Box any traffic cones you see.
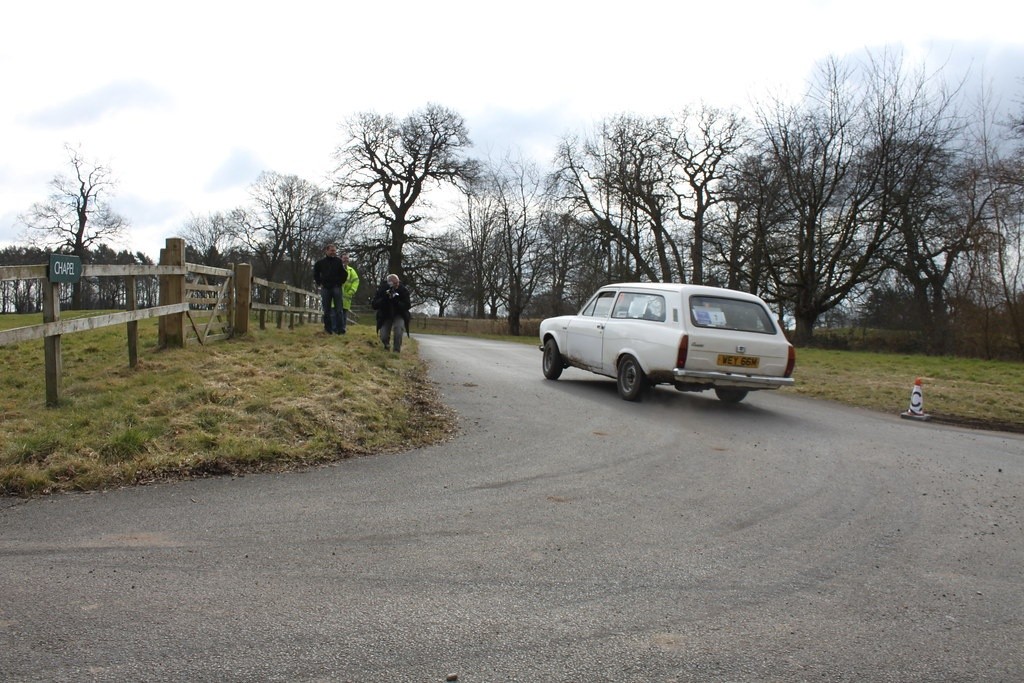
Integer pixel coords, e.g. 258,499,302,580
901,379,932,422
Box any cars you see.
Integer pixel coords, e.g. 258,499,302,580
538,282,799,403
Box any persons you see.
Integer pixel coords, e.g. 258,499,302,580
314,245,348,335
372,273,410,353
330,253,359,330
